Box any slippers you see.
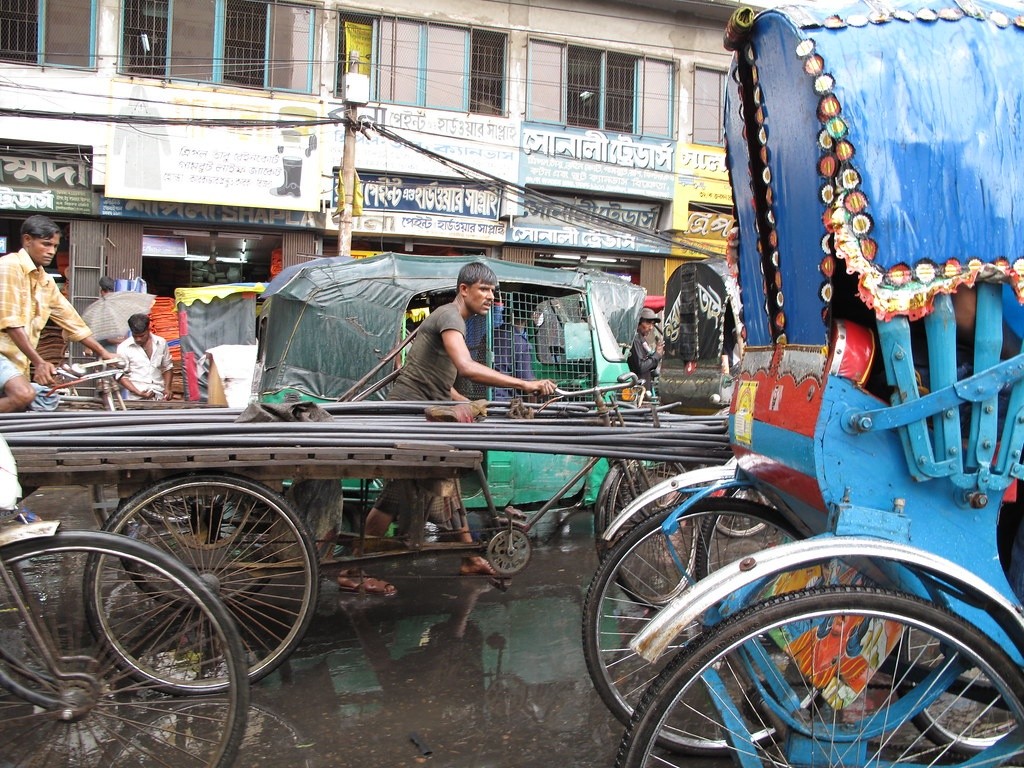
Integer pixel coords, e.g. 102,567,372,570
460,560,497,576
14,507,37,523
339,577,398,595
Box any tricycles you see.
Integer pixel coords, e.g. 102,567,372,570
30,0,1024,767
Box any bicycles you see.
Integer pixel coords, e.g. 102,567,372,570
0,505,252,768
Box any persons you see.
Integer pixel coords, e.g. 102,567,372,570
339,261,557,597
0,215,174,413
626,307,665,392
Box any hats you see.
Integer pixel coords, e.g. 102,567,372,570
642,307,661,324
